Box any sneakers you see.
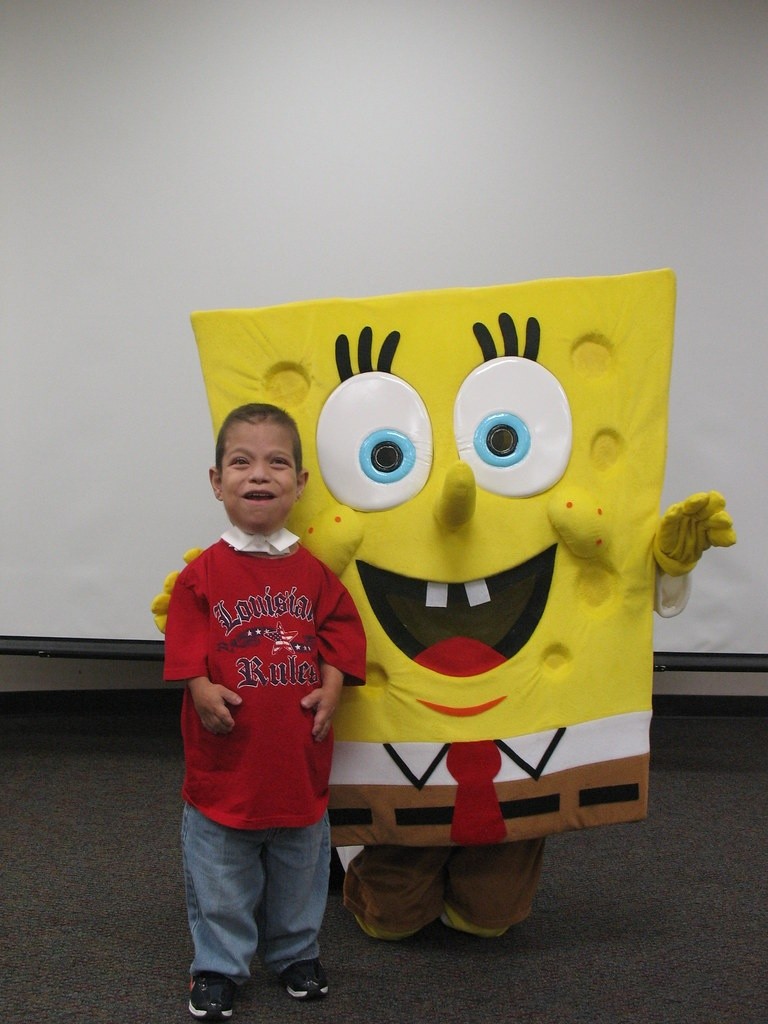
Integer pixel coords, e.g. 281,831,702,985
280,958,329,999
189,972,236,1021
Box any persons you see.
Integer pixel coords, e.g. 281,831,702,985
163,404,367,1018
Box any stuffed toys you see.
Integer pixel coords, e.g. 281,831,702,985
153,268,737,941
